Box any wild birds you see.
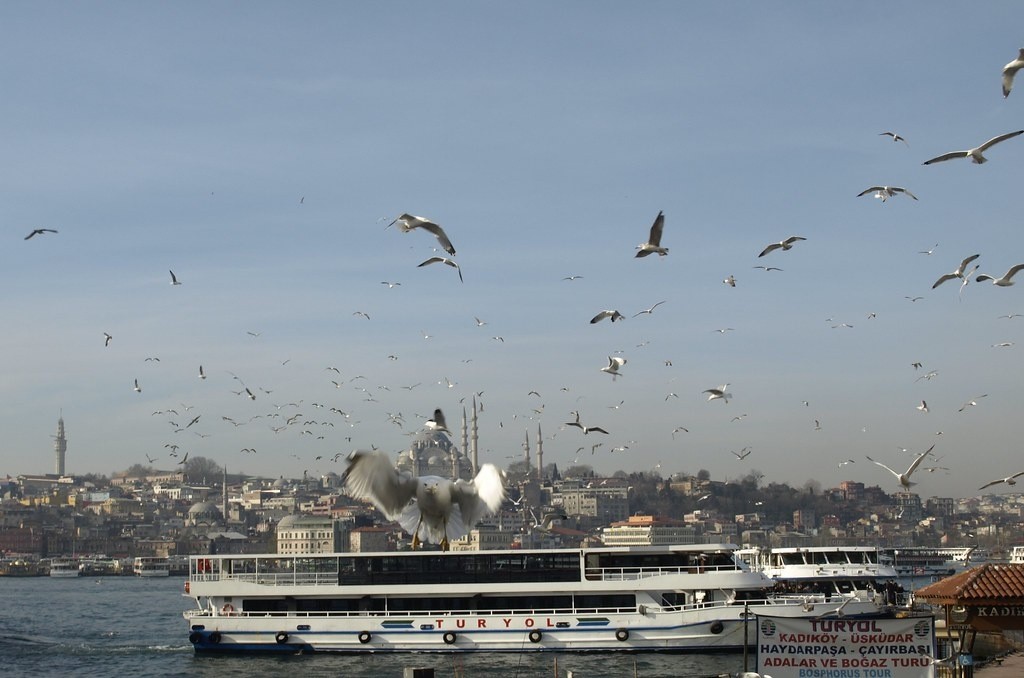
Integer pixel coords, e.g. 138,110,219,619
815,594,857,620
945,545,978,571
104,48,1024,551
23,228,59,240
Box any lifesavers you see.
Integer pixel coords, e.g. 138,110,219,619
616,628,629,642
710,620,723,634
222,604,234,615
357,630,372,644
275,631,287,644
188,631,204,644
198,560,211,572
529,629,542,643
207,632,221,644
443,630,458,645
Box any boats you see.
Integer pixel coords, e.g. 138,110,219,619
181,542,978,659
0,553,248,579
